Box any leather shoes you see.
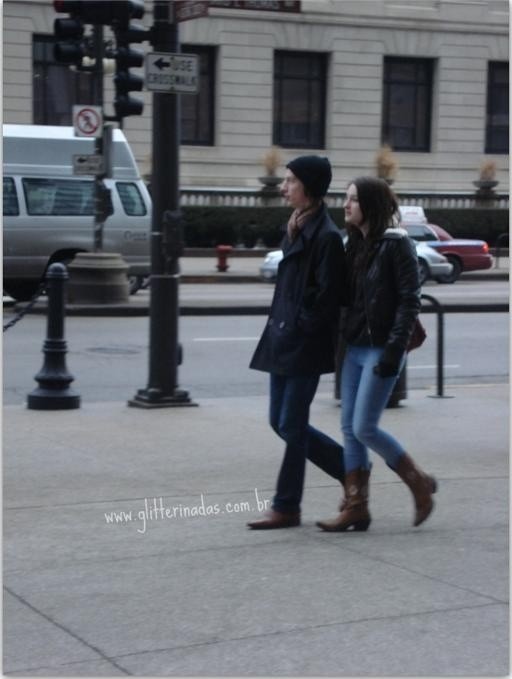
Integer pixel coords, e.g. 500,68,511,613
248,511,300,529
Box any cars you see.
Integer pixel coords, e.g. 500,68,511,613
258,224,454,285
397,205,493,284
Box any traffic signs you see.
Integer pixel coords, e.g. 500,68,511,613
143,52,197,94
72,151,109,178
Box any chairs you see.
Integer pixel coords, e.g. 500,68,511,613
51,189,109,215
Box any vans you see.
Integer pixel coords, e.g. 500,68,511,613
2,120,156,300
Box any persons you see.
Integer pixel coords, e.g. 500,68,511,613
316,177,437,531
244,155,373,529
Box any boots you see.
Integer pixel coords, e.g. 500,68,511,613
317,471,370,530
389,453,435,526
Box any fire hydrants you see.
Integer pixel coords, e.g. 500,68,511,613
215,245,232,272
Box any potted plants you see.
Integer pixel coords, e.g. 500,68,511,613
375,142,397,187
472,157,500,194
261,147,286,191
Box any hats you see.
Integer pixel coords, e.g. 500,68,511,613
287,156,331,197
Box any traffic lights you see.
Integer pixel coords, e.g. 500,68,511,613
114,0,149,117
52,1,84,68
